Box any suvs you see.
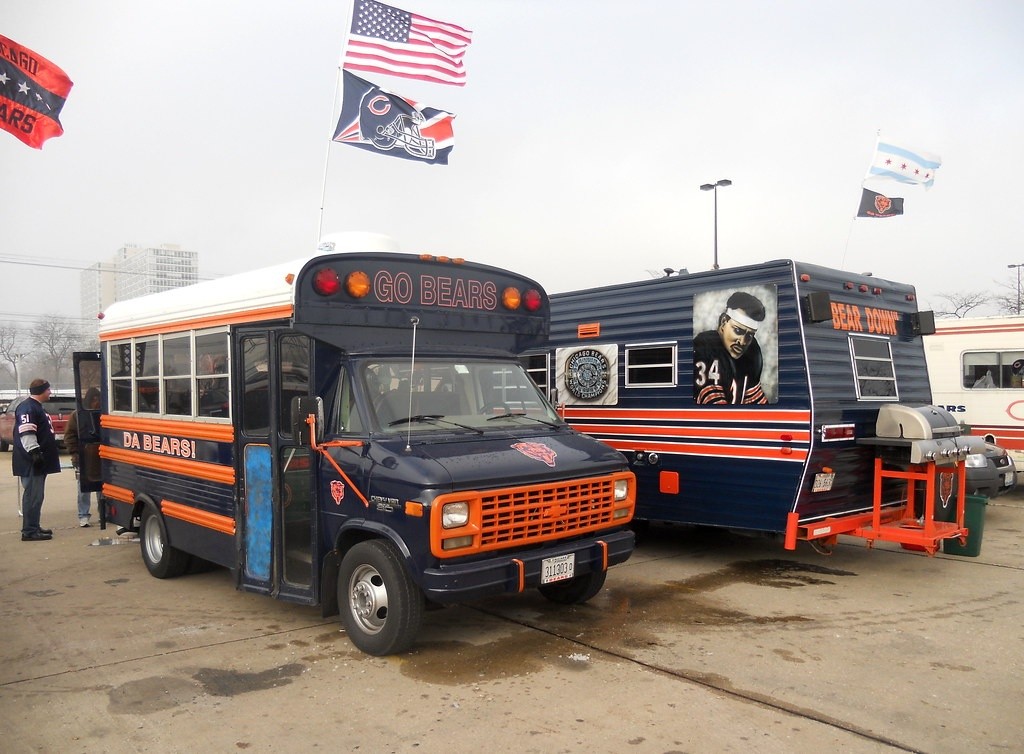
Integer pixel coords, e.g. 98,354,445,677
0,393,77,452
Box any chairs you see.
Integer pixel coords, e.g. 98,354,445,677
372,388,419,433
427,376,464,418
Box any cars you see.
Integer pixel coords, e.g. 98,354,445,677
955,436,1018,501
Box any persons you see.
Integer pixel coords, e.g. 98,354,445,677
64,388,101,527
12,379,61,541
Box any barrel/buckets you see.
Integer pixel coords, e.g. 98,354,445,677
944,495,988,554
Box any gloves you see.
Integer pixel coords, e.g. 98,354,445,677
32,451,45,466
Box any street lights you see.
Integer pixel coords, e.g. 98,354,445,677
700,179,731,270
1008,262,1024,315
15,354,25,398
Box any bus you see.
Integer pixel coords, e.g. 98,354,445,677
69,251,639,656
389,258,984,553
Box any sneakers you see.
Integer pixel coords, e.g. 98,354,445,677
79,517,90,527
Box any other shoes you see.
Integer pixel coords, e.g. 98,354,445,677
22,526,53,541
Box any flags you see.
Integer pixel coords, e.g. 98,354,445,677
0,35,74,151
866,134,943,192
341,0,472,87
330,68,456,165
856,188,905,218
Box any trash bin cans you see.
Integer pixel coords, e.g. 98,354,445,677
943,494,987,558
